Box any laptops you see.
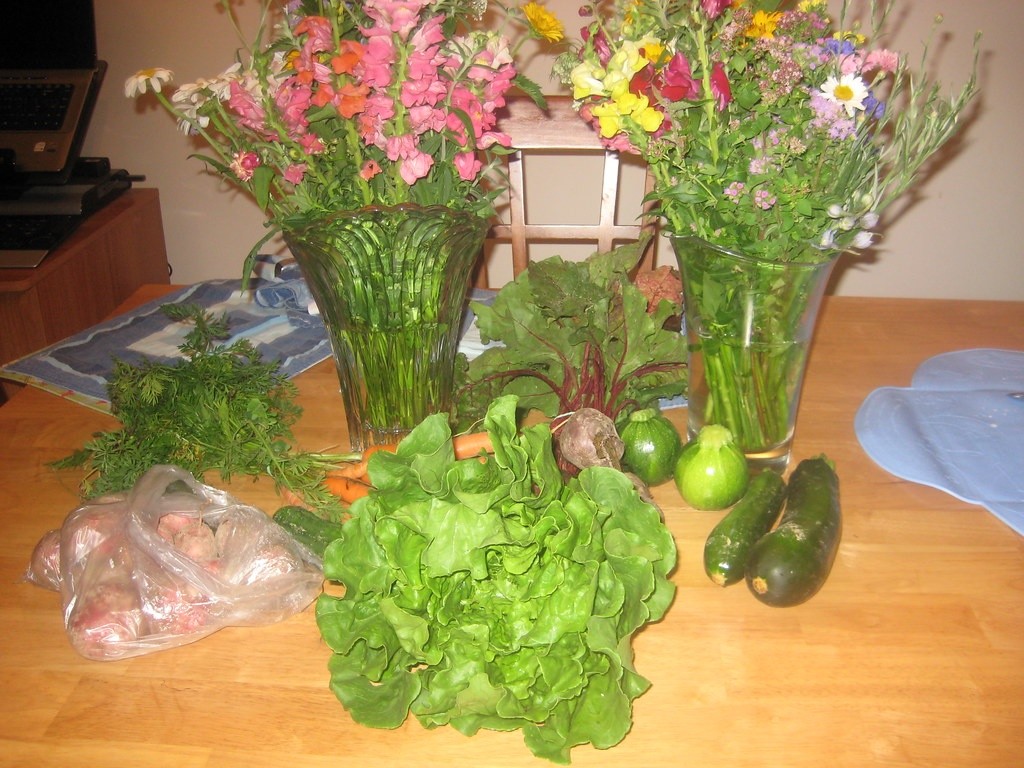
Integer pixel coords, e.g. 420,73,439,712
0,0,99,172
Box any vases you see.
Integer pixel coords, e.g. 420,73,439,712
283,204,491,458
669,235,842,475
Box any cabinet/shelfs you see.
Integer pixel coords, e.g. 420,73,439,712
0,188,171,405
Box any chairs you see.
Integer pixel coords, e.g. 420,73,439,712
460,95,662,289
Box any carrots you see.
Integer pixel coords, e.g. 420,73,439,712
360,430,495,461
323,459,369,479
318,475,372,504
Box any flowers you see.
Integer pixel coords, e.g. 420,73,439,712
122,0,565,435
550,0,995,453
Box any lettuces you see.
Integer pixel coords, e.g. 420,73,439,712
313,391,677,766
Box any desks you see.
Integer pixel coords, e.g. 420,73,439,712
0,281,1024,768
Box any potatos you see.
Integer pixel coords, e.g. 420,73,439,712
26,494,300,661
